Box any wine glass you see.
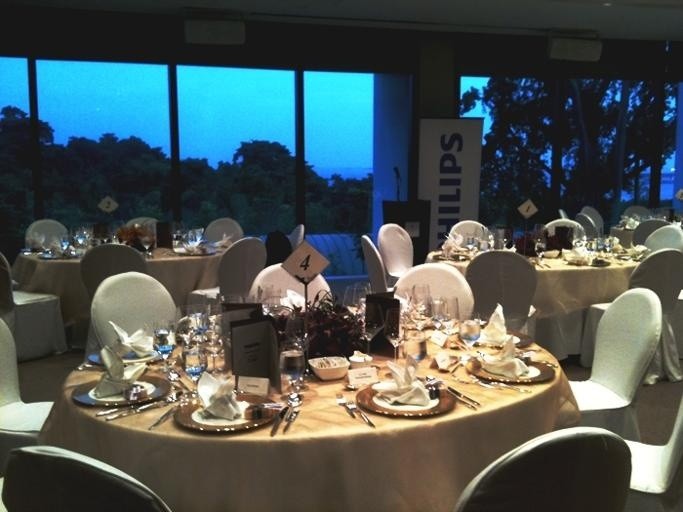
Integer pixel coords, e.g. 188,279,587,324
534,237,545,263
605,234,614,255
151,281,482,407
466,234,475,252
60,224,204,258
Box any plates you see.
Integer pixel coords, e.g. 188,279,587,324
71,330,559,437
